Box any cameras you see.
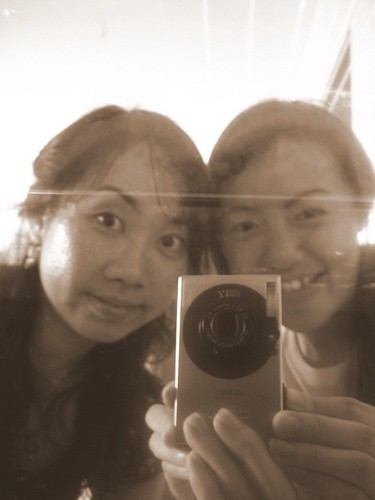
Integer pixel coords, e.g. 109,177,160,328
173,274,283,445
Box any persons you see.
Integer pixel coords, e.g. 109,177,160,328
1,105,298,499
146,100,375,500
